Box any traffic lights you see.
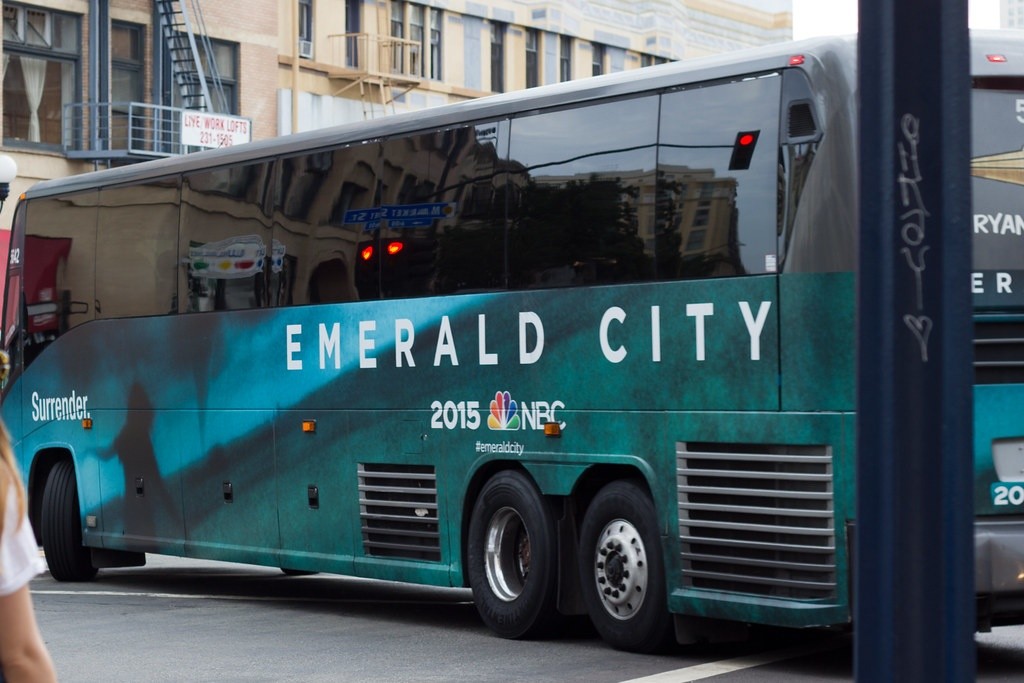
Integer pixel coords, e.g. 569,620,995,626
728,129,761,171
358,237,412,270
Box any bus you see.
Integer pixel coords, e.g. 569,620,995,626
0,30,1024,654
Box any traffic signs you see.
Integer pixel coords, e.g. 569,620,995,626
341,200,458,231
26,300,90,316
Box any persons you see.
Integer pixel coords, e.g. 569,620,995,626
0,397,65,683
